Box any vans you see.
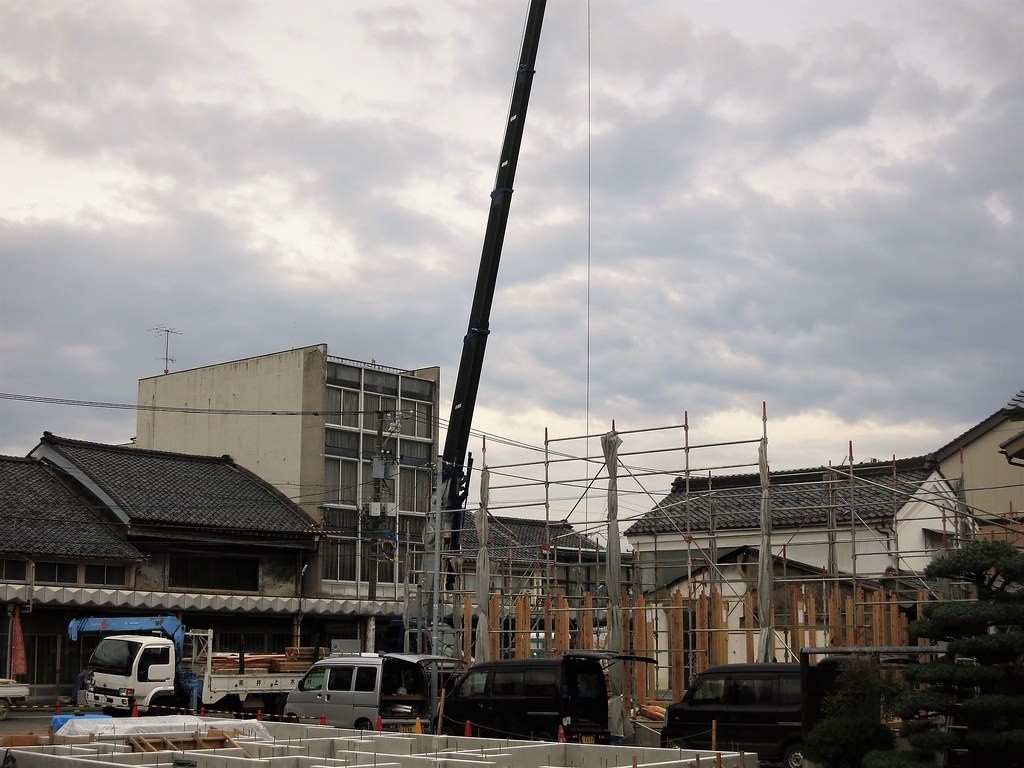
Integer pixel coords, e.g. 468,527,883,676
429,650,659,745
660,660,863,768
282,651,469,733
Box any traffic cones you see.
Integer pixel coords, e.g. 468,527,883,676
256,710,263,721
558,725,567,743
414,717,423,734
131,702,139,717
374,715,383,732
200,707,205,716
53,701,61,715
465,720,473,737
319,714,327,726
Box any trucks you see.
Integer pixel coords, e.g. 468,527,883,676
0,684,30,720
67,615,321,716
